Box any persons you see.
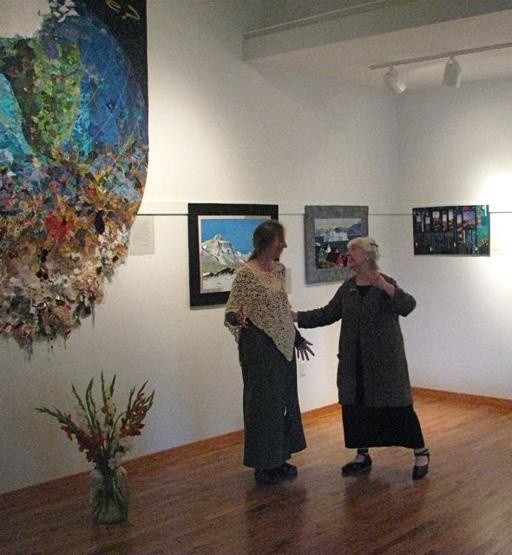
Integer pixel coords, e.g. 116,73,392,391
224,219,316,485
291,236,431,480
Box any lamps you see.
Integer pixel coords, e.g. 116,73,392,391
382,56,464,95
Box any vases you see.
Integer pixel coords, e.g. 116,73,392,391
88,462,129,525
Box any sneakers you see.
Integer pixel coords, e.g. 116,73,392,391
412,448,430,480
342,448,371,474
255,462,297,484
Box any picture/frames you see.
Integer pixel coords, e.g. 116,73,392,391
305,205,370,284
411,204,491,256
187,201,279,308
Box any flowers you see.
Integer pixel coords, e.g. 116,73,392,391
33,367,155,519
